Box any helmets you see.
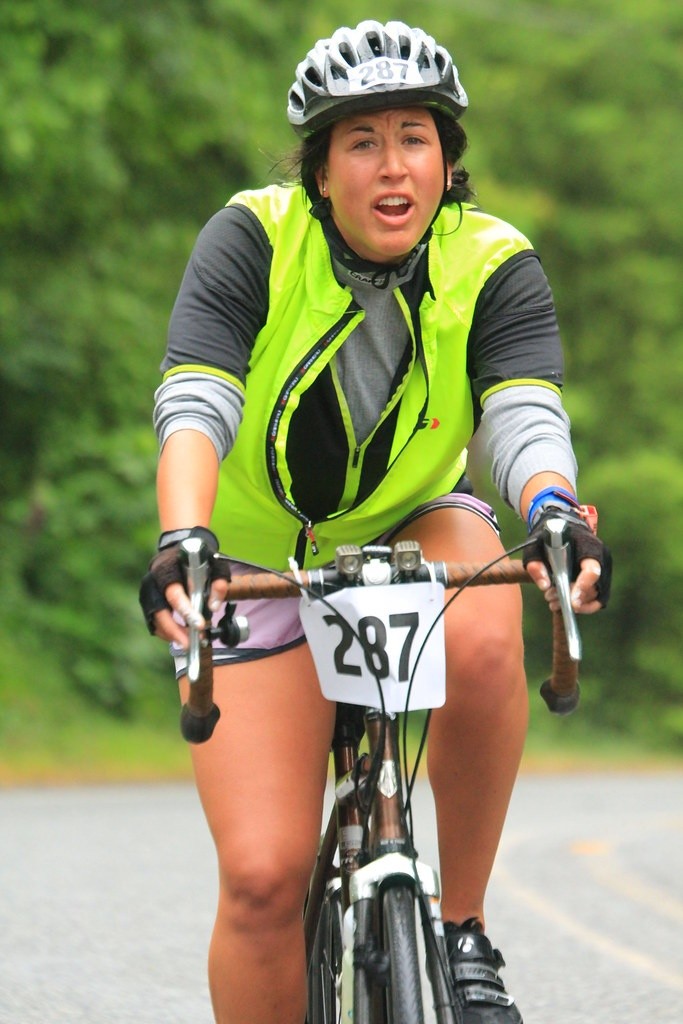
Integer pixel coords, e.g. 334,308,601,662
287,19,468,142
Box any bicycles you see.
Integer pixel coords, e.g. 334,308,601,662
179,516,585,1024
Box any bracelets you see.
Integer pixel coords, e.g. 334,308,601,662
529,484,581,533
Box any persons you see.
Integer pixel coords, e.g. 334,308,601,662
140,22,612,1024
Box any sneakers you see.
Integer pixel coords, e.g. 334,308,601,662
445,916,524,1024
